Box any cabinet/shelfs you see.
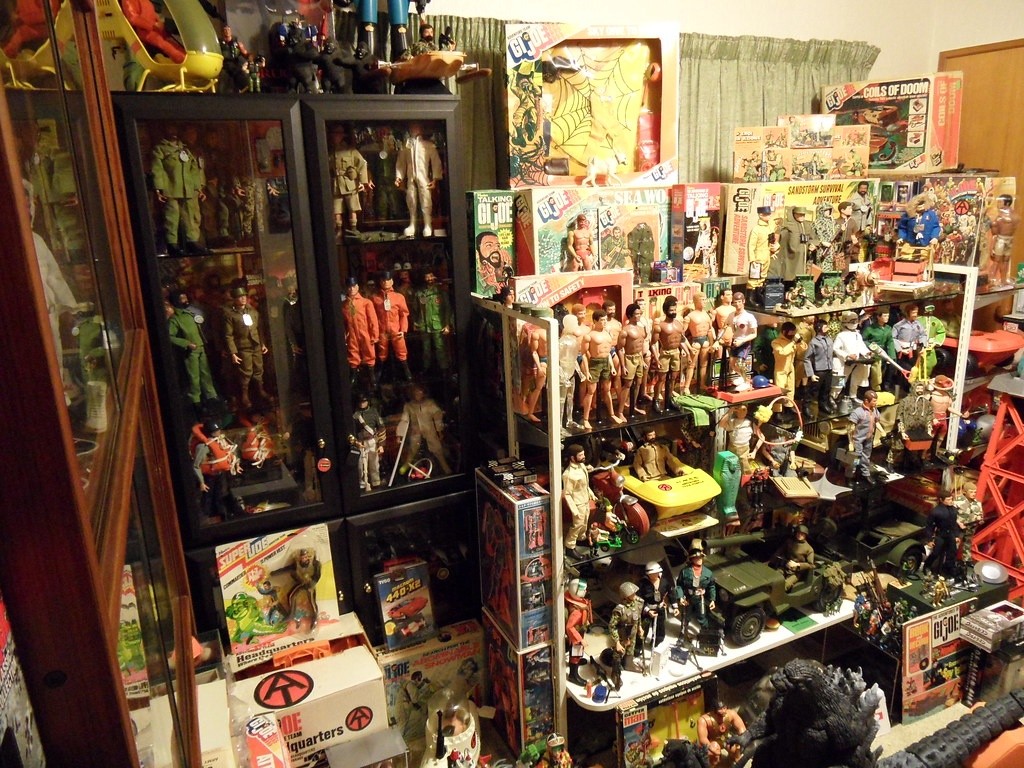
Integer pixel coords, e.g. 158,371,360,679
110,88,487,645
0,0,202,768
469,263,1024,768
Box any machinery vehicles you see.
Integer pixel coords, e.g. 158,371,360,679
691,506,928,647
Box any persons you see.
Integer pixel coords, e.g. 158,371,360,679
166,276,273,521
341,260,454,489
218,0,453,92
505,181,1024,768
155,125,282,252
330,121,443,242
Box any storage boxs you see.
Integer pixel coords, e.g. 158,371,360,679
105,24,1023,768
